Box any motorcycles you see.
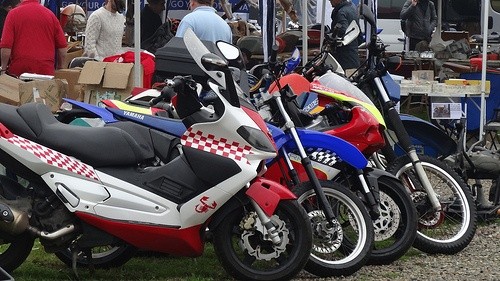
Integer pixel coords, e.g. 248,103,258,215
0,2,479,281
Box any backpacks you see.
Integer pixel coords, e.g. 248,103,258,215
400,2,432,32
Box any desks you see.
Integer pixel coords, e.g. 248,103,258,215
397,92,489,151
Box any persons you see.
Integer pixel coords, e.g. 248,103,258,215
0,0,67,79
400,0,437,51
175,0,233,45
329,0,360,70
140,0,166,42
84,0,126,62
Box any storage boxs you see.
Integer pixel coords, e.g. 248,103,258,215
390,70,491,97
0,41,154,113
